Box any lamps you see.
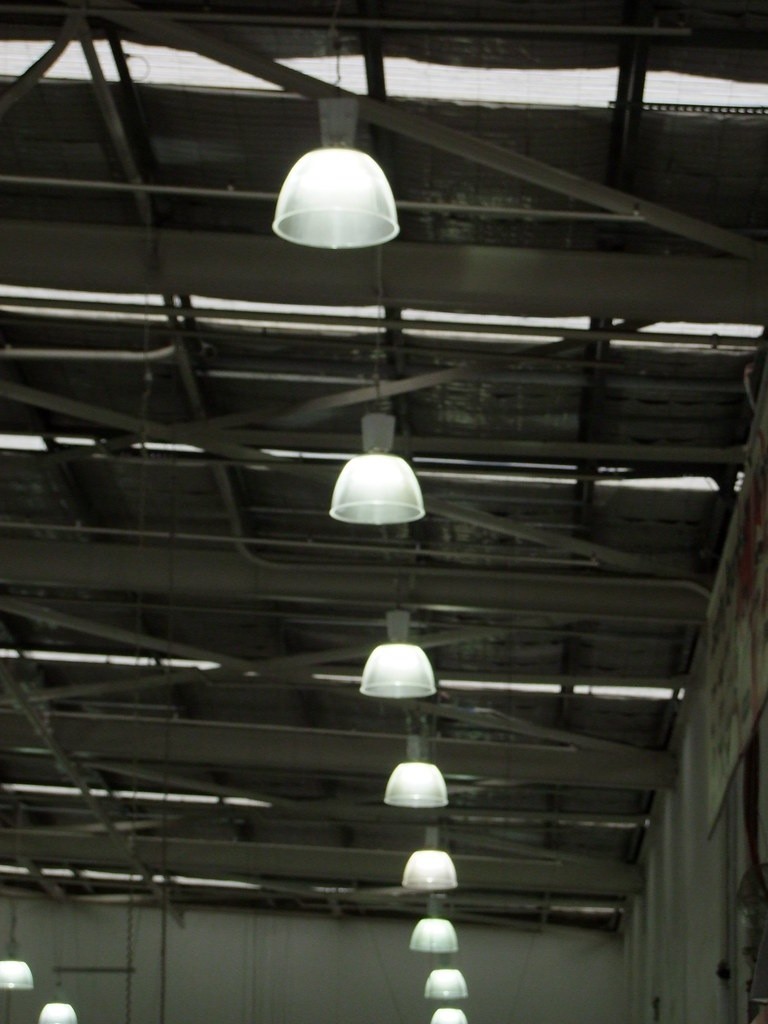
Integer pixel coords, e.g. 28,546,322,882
410,897,459,953
273,99,400,249
329,413,426,524
430,1008,467,1024
360,610,436,698
39,984,77,1024
424,954,469,1001
0,898,34,990
384,734,449,807
402,827,459,891
